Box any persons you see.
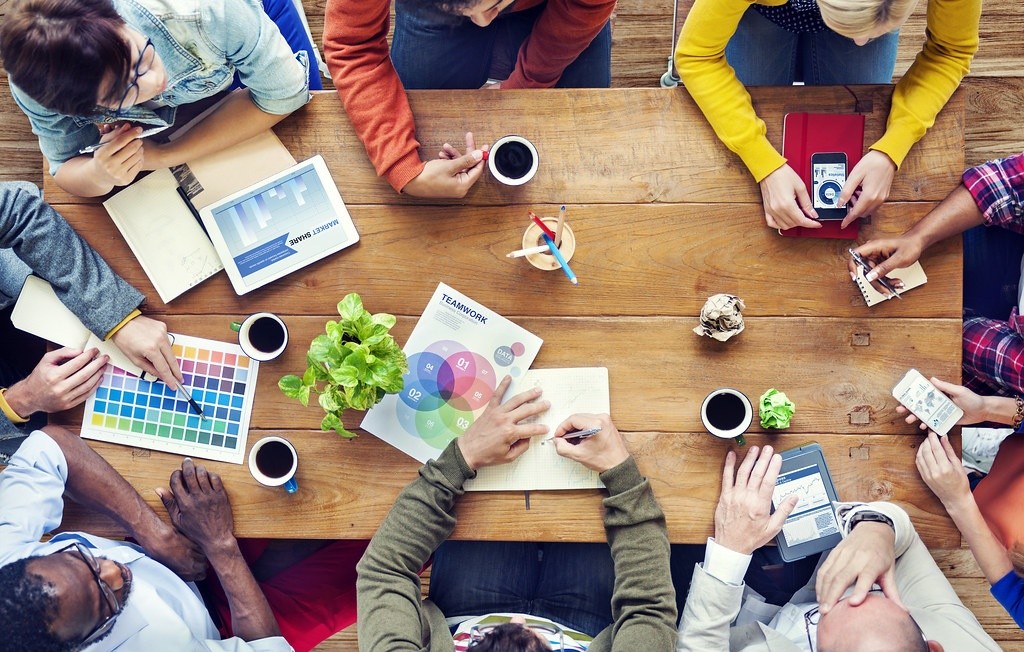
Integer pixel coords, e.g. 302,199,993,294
0,0,322,198
0,424,297,652
0,185,185,465
677,445,1003,652
356,375,678,652
673,0,982,231
896,378,1024,630
847,150,1024,399
322,0,617,199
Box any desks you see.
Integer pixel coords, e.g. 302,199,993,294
38,84,967,550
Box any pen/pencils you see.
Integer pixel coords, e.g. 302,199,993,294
506,245,549,258
547,427,602,441
542,233,578,286
528,211,555,239
173,376,207,422
79,126,169,154
554,206,565,247
849,248,903,300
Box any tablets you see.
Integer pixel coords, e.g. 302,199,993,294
199,155,359,295
767,443,844,560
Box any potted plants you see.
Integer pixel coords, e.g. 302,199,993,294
278,292,405,437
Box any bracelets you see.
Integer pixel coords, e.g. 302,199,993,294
1012,395,1024,428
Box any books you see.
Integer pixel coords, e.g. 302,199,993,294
102,127,300,306
855,260,928,307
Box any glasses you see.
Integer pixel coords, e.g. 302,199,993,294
466,623,564,652
83,14,155,118
804,589,929,652
47,542,119,651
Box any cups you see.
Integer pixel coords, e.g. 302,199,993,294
701,388,754,447
229,311,288,359
483,135,540,186
248,435,298,494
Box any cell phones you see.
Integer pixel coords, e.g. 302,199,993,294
892,368,965,436
810,151,851,221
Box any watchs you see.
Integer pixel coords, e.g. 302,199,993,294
850,510,893,531
